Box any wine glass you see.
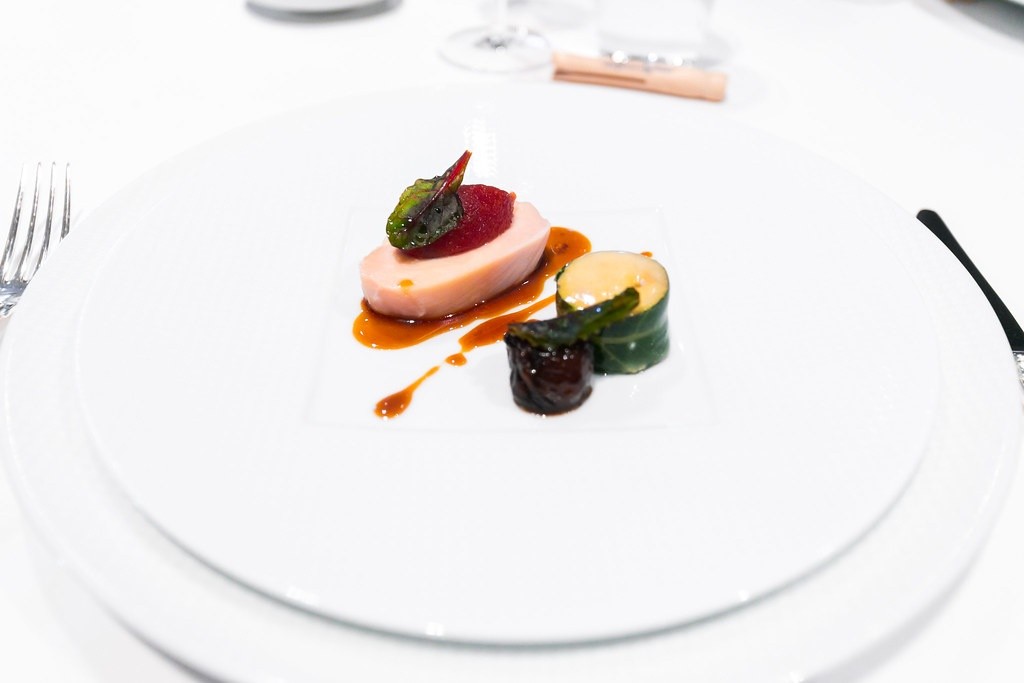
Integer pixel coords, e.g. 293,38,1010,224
440,0,551,70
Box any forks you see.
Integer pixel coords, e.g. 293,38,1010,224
0,161,73,325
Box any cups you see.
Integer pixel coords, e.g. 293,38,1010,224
598,0,728,64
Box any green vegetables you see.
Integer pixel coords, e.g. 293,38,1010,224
386,150,473,249
509,285,640,349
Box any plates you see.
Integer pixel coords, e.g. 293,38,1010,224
0,83,1024,683
